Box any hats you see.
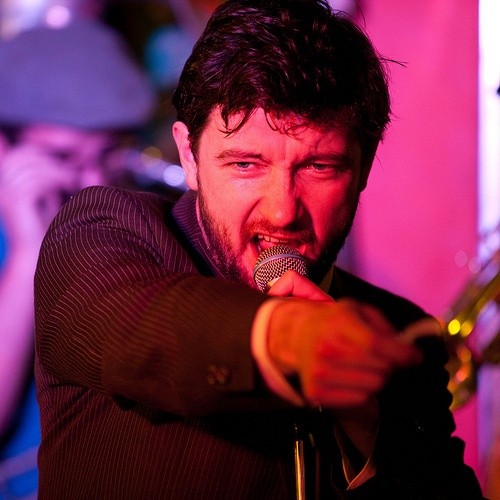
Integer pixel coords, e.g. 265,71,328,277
0,22,155,130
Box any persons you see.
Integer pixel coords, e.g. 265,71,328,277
0,10,154,500
34,1,486,500
